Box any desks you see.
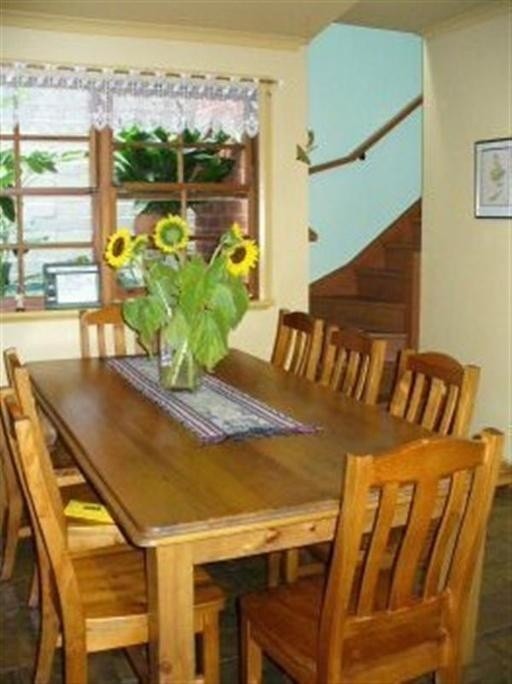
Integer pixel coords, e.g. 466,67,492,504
21,347,512,684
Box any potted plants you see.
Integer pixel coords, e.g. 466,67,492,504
114,129,240,243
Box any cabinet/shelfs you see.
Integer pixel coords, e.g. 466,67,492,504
0,128,107,316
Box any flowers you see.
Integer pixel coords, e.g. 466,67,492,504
106,215,260,374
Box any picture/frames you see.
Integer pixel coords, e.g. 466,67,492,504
473,135,510,219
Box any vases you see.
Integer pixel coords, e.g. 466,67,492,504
153,326,213,394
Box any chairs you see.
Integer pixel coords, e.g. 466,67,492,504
287,347,483,590
0,384,228,684
268,306,328,384
262,323,390,589
2,346,125,610
235,426,508,684
75,305,159,362
